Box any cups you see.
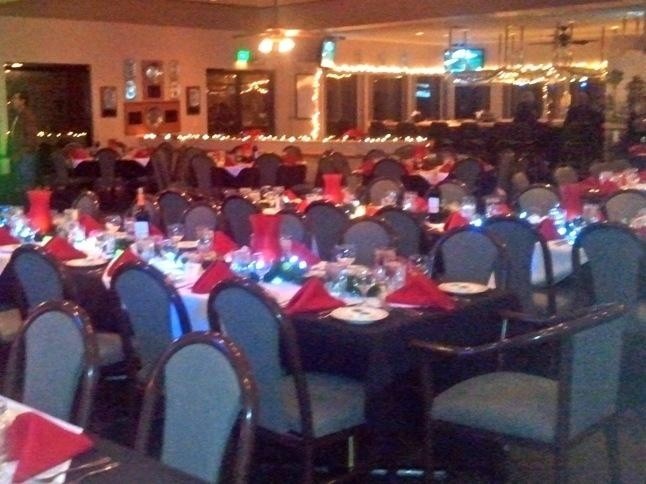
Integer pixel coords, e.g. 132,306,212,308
383,257,407,288
409,254,435,280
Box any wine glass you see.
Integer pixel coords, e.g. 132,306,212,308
460,196,476,221
334,243,357,265
104,215,122,253
167,223,185,251
249,252,275,282
352,266,377,295
158,239,179,262
123,217,138,239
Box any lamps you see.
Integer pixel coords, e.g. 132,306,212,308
257,27,299,54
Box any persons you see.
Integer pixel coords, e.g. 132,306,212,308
9,90,39,206
564,92,605,131
514,91,546,128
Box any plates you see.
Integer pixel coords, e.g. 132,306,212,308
437,281,488,295
331,306,390,324
66,257,107,269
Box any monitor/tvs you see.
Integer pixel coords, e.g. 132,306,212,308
442,46,485,73
317,38,336,69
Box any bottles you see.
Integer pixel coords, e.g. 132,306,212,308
427,170,441,223
134,187,150,239
252,145,259,161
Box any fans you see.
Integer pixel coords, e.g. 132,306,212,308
232,0,319,39
528,26,597,49
